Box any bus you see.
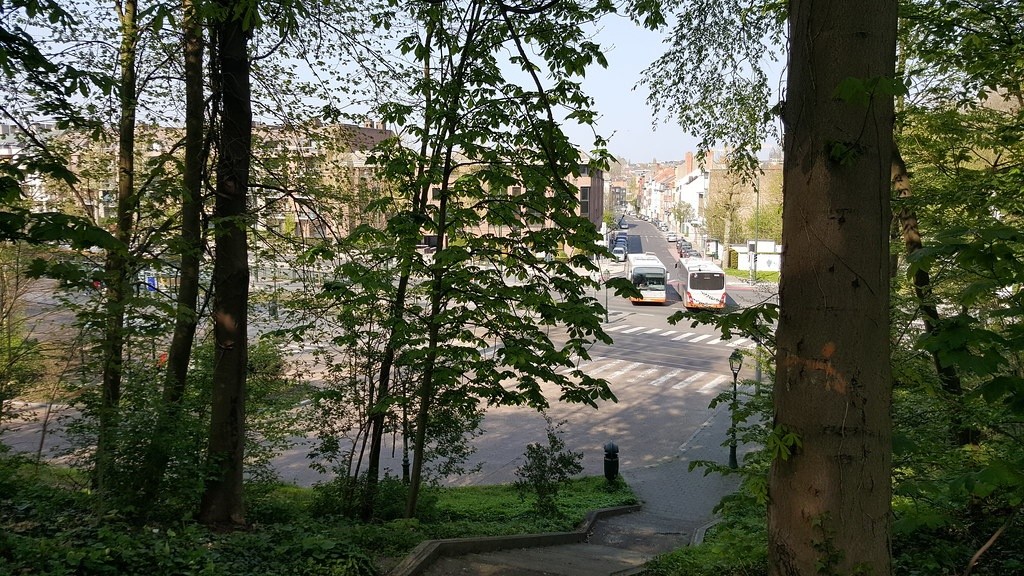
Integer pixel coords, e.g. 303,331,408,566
674,255,726,309
624,252,668,303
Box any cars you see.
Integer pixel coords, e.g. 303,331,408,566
610,218,630,262
636,212,702,257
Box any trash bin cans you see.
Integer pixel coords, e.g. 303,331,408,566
603,440,619,480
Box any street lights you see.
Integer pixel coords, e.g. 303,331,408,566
729,348,744,469
602,268,611,324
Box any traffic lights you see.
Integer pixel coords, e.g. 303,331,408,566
754,253,757,262
